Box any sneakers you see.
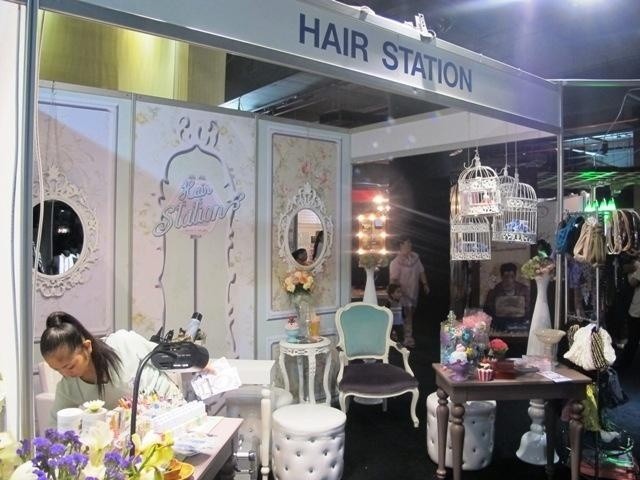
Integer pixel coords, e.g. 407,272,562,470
390,342,416,351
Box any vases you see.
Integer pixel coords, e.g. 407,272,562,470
526,276,558,355
293,290,312,339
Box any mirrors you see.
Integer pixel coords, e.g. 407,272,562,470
279,181,335,278
30,163,99,300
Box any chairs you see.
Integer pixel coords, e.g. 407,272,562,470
335,302,421,428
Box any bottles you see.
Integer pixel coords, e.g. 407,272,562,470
186,312,203,342
439,309,463,366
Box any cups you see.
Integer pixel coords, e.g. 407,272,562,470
312,315,320,337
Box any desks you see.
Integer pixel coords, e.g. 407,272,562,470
175,413,243,480
432,357,593,480
279,336,332,408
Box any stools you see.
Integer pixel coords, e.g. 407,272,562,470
426,391,497,471
272,404,348,480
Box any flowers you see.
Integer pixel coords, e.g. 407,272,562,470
520,252,557,282
16,398,175,480
281,271,318,297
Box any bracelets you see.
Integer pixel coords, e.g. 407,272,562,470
422,282,428,287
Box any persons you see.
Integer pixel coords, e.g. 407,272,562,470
480,262,532,359
383,283,406,360
311,230,324,261
352,267,387,297
531,238,566,330
38,308,221,437
386,233,432,349
33,201,85,276
605,234,637,350
621,253,640,346
291,248,309,267
